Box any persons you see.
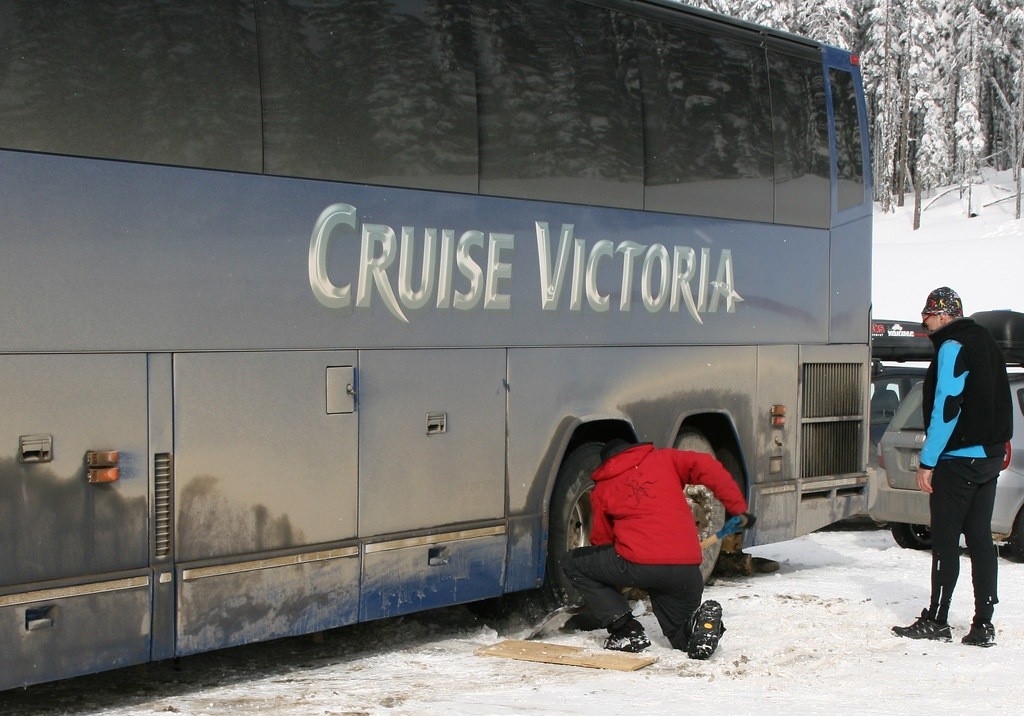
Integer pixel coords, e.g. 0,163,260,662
561,439,755,661
890,286,1014,647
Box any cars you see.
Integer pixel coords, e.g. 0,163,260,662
868,364,929,467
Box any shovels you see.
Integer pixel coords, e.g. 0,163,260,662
524,515,749,642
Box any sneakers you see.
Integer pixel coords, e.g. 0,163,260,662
604,626,651,653
961,623,997,647
891,608,952,643
688,600,726,659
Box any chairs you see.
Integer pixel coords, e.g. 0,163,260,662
868,389,900,424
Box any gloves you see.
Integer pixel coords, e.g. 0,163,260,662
1001,441,1011,470
737,513,756,529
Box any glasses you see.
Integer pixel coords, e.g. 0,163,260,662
922,316,932,328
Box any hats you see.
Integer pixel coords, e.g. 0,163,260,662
921,287,963,318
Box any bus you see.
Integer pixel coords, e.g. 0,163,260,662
0,2,875,693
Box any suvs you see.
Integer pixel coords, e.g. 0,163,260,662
868,367,1024,554
